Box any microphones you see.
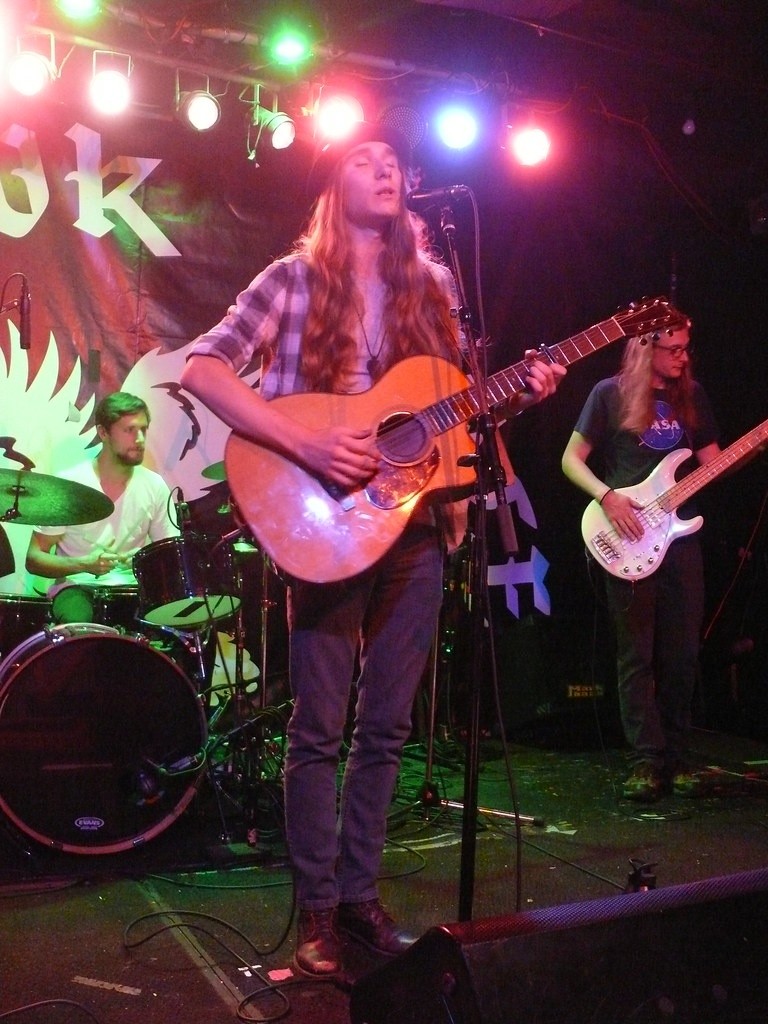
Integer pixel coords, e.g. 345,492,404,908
19,277,31,349
405,184,467,212
177,489,184,526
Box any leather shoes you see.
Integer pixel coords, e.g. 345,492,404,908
338,897,420,958
292,907,344,980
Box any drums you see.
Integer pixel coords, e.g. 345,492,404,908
82,581,142,628
0,622,211,855
209,527,279,598
131,533,245,627
0,592,54,660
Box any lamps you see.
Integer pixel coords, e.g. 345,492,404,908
168,67,222,134
375,93,427,150
245,83,297,150
7,28,58,97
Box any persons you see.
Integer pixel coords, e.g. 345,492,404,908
25,393,181,626
559,294,725,800
181,129,570,976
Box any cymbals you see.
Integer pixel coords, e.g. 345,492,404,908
201,459,228,482
0,468,116,526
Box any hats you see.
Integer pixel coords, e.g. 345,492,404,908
308,126,413,197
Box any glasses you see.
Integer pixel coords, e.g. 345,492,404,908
654,342,698,358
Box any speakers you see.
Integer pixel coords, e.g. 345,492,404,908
348,867,768,1024
486,614,621,735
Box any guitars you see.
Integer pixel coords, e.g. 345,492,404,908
222,293,693,591
580,419,768,583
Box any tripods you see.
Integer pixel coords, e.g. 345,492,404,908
387,618,549,828
190,601,291,808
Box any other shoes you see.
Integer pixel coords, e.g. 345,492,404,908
624,762,663,799
665,763,712,797
